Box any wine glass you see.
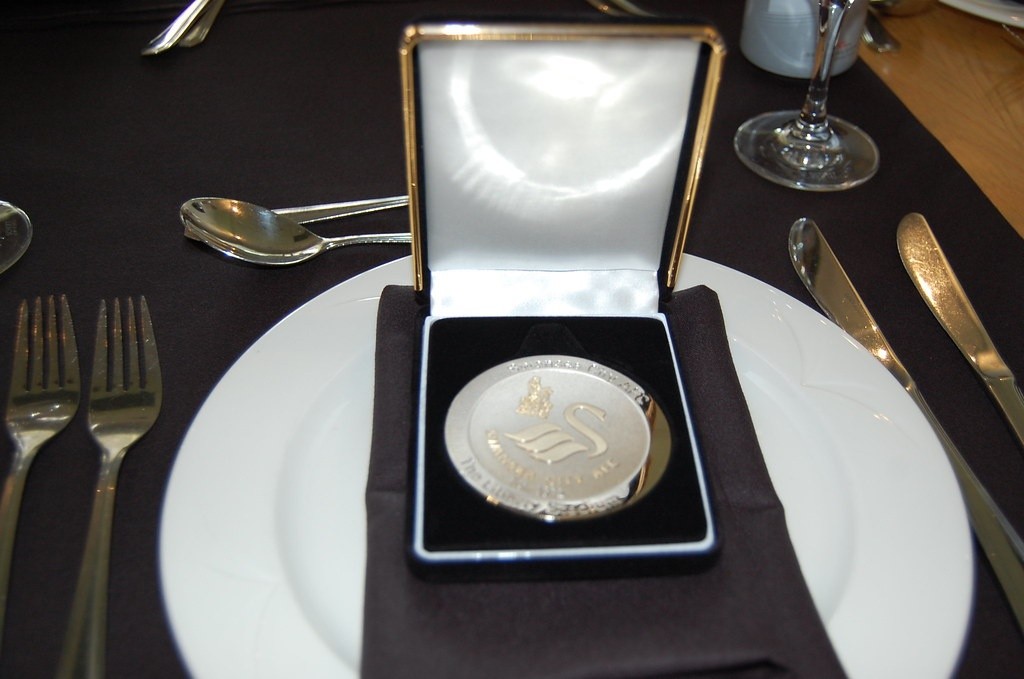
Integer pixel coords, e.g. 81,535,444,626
734,0,880,192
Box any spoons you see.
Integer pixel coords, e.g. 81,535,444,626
181,197,414,266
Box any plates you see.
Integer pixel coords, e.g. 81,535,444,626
160,252,973,679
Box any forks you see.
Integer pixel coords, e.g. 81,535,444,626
60,297,162,679
0,294,82,638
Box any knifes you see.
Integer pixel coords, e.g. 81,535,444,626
788,217,1024,629
896,213,1024,446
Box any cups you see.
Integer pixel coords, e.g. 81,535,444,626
740,0,869,79
